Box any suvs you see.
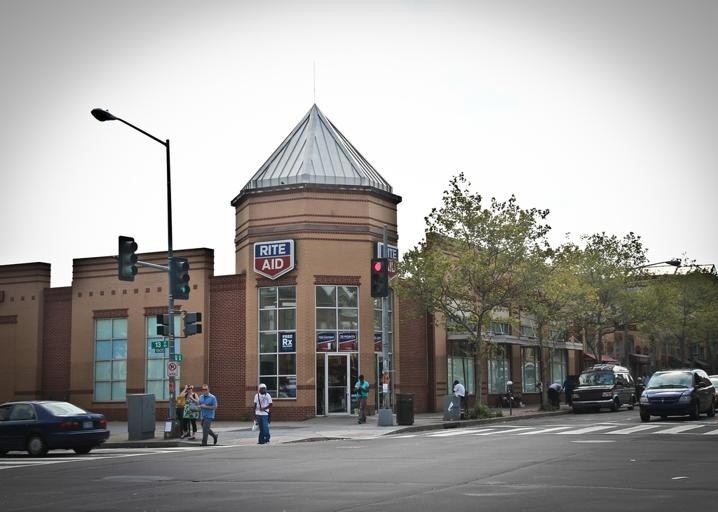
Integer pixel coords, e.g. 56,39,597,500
568,363,640,411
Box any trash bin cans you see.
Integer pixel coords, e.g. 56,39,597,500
442,394,462,420
396,393,416,426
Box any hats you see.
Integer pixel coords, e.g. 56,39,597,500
258,383,267,393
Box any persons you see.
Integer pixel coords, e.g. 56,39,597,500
453,380,465,403
642,370,650,386
198,383,218,446
180,383,200,441
549,383,565,407
353,374,370,424
253,382,273,444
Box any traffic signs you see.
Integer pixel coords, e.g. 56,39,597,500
151,340,168,349
173,353,183,361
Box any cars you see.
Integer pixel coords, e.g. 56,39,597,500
259,372,297,398
634,368,718,420
0,399,111,455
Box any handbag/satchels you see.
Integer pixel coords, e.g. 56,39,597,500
183,399,199,420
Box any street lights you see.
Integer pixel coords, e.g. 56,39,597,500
91,105,184,443
622,259,683,371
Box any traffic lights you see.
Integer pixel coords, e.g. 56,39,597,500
155,311,170,337
183,309,204,337
118,234,139,282
172,255,190,299
370,257,389,297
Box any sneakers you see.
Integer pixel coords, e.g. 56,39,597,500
181,433,218,446
